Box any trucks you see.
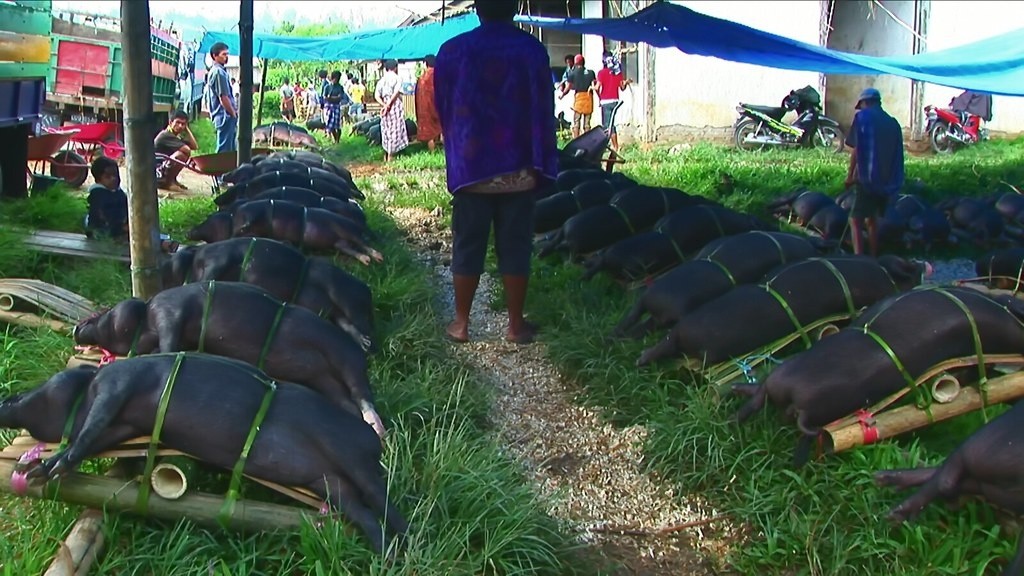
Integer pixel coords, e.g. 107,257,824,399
45,1,184,137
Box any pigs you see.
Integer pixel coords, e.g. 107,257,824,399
0,352,411,557
533,167,1024,531
183,120,387,265
71,278,388,438
162,237,374,353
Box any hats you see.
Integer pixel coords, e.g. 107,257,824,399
854,88,881,110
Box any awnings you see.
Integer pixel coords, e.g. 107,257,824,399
195,0,1024,96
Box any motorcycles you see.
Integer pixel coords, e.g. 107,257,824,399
732,85,845,156
924,90,993,153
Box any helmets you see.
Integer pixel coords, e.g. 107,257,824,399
782,94,799,111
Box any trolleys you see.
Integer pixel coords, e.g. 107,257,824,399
24,128,94,186
60,122,126,159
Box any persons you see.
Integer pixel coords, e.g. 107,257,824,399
433,0,559,345
374,59,408,162
153,110,198,192
562,54,576,81
293,81,319,119
279,77,295,124
84,156,180,254
415,54,442,153
841,87,905,258
318,69,365,145
588,51,630,152
558,54,596,138
208,42,238,189
